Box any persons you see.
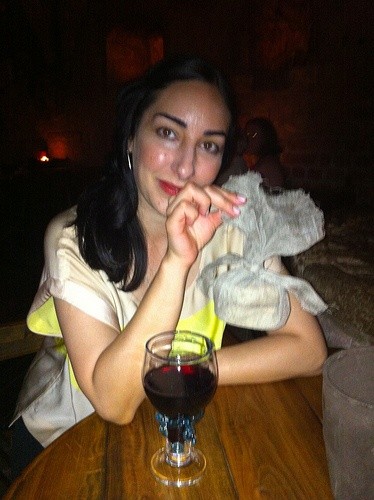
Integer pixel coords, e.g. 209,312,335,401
6,52,335,491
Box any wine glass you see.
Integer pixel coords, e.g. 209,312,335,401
142,328,219,484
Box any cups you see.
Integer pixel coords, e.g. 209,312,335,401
317,346,373,499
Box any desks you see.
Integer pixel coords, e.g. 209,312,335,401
4,345,350,499
